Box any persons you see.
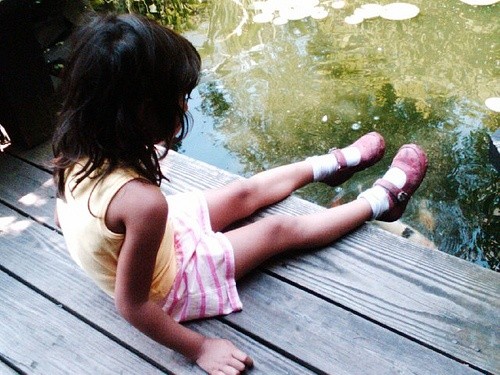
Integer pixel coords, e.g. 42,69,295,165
42,9,431,374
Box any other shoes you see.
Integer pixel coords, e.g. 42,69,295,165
328,132,385,186
374,143,428,223
